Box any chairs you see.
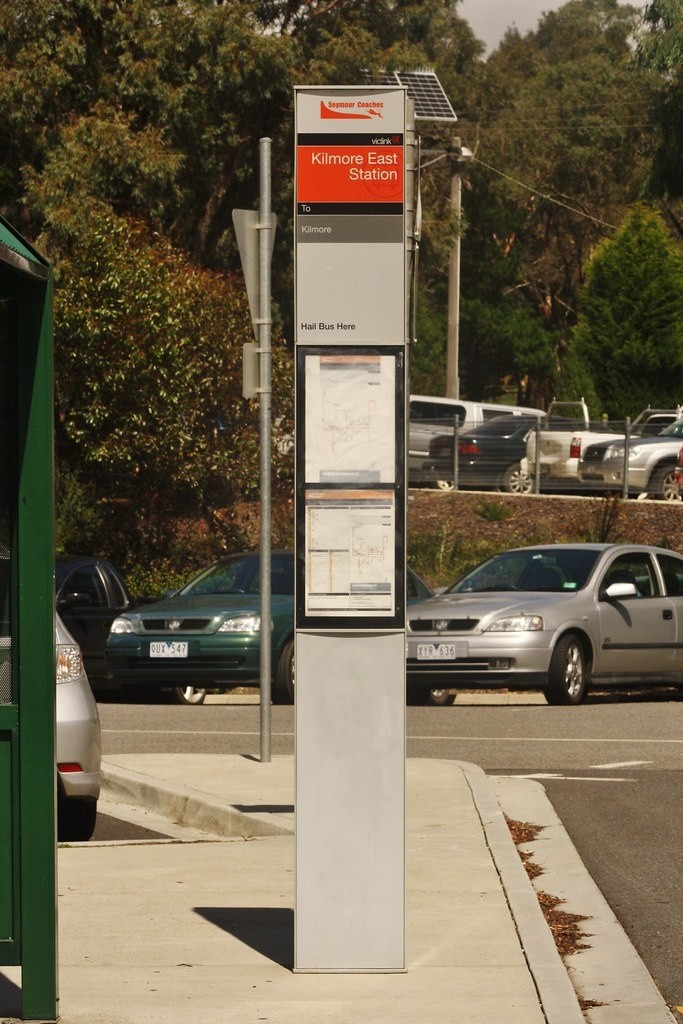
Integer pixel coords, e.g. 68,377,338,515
606,571,639,587
518,566,566,590
272,571,290,594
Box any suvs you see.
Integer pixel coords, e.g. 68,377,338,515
407,393,550,493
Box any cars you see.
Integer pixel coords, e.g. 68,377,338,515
404,543,683,704
0,554,147,708
0,555,103,842
104,549,436,703
429,412,683,503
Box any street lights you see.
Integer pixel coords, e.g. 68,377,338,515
404,146,474,387
